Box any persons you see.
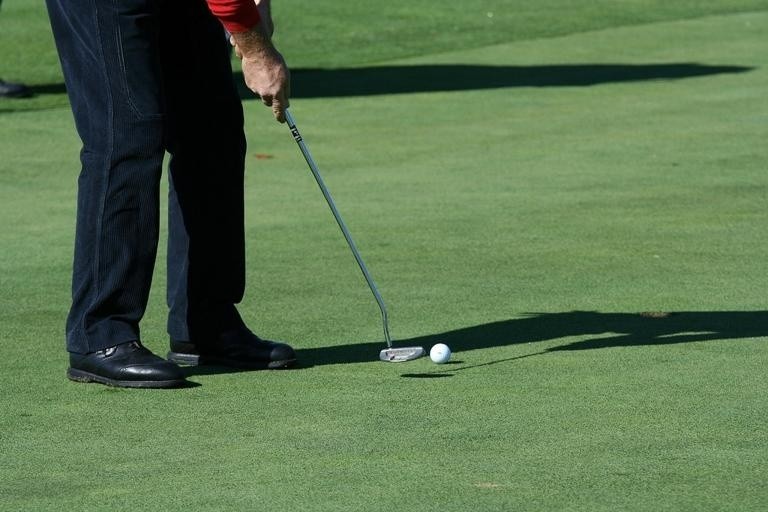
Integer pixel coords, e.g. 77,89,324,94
43,1,299,387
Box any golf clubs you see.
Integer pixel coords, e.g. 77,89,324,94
285,110,424,362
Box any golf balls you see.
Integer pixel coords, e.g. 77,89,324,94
430,343,450,364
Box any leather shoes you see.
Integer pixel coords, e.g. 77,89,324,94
66,341,186,389
167,332,296,369
